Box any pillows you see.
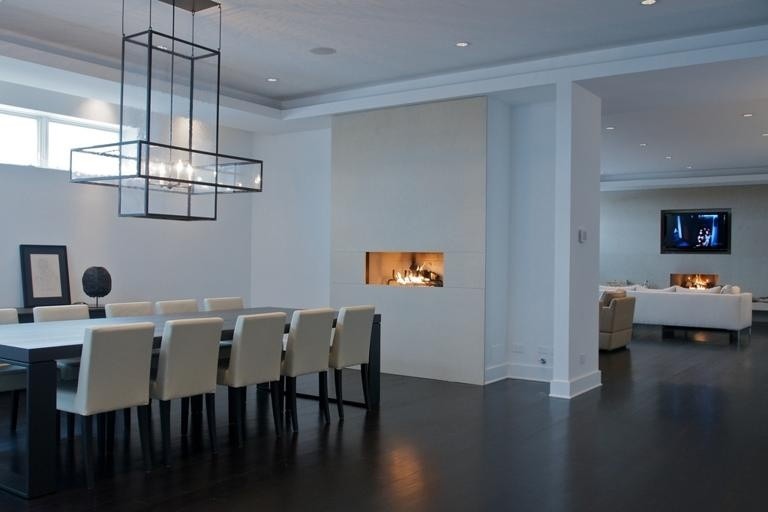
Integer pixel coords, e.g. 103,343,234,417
599,284,740,294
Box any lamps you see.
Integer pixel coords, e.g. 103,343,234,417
68,2,263,223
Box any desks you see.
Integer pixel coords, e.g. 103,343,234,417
1,307,381,499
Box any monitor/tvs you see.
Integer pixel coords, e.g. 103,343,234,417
661,208,732,255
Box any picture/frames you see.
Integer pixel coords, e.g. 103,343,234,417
19,244,71,308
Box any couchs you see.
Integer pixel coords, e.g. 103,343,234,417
627,290,753,344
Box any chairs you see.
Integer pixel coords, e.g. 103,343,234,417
599,288,635,351
